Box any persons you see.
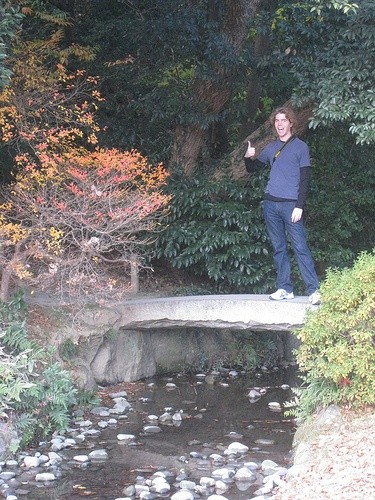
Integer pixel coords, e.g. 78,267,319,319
245,107,328,304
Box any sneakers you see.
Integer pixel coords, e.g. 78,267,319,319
308,290,324,305
269,289,294,300
